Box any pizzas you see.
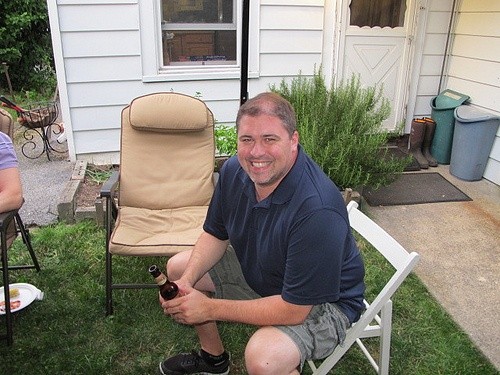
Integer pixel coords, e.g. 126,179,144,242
0,300,20,311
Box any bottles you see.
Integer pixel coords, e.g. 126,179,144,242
149,265,181,300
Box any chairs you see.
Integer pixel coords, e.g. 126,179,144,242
100,91,228,316
306,200,421,375
0,198,40,347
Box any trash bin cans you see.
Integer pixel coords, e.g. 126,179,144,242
430,89,471,164
449,105,500,182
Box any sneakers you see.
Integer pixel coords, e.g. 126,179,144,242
160,349,231,375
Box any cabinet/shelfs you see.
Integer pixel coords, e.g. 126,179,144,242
173,31,216,58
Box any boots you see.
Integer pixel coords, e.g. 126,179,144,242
422,117,438,167
410,118,429,169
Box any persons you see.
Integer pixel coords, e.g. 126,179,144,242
0,131,23,213
158,92,365,375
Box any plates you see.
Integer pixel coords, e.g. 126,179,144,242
0,282,39,314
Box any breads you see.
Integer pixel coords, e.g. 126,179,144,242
10,289,20,298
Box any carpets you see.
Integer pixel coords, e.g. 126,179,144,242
370,148,421,170
366,172,473,207
